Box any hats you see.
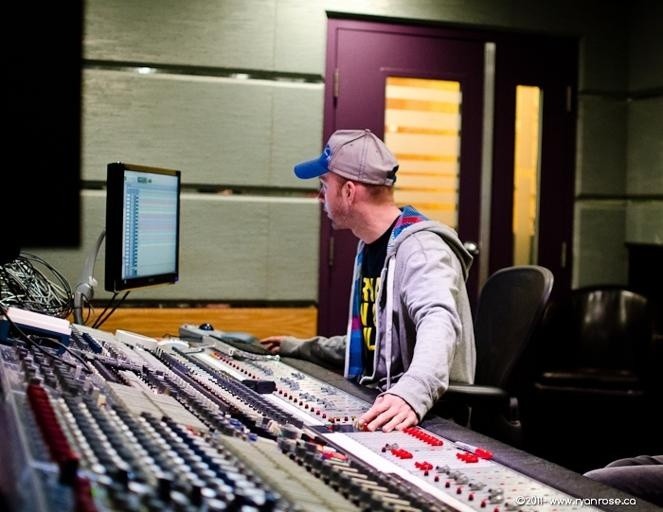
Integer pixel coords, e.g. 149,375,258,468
294,129,399,187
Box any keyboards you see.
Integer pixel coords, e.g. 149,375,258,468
201,333,281,361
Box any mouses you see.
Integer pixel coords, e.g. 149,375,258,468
223,334,256,345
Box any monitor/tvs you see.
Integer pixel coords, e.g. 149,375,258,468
104,162,181,291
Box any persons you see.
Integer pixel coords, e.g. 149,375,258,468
255,125,478,434
